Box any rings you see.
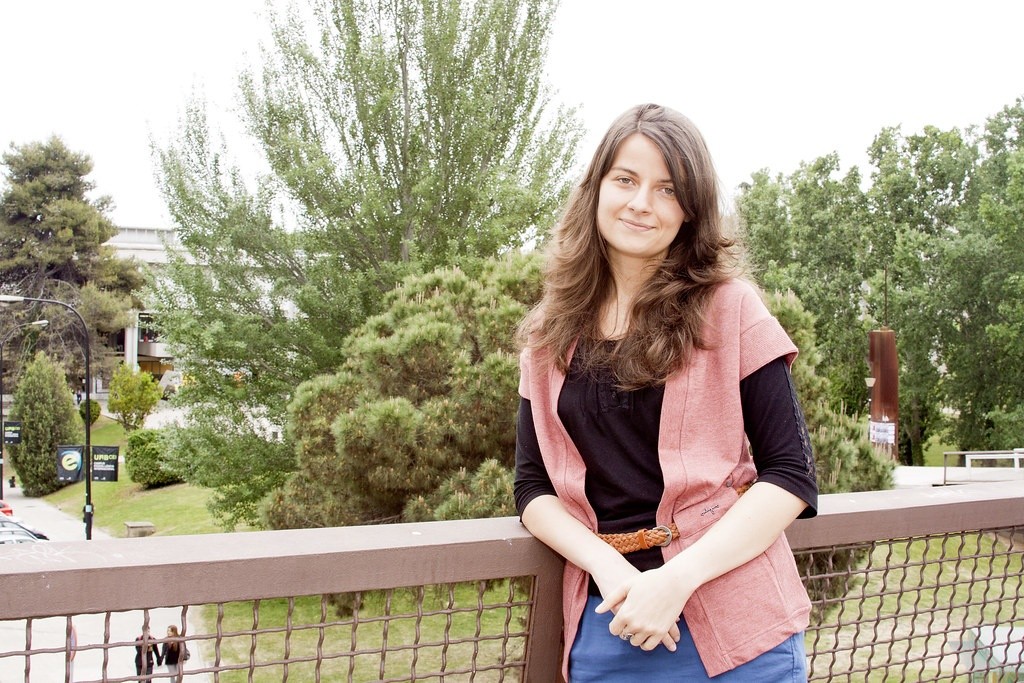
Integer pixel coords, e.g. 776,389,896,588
622,629,634,640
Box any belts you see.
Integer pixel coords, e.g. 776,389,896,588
582,482,757,552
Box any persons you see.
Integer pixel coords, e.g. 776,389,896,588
135,625,162,683
511,103,817,683
158,625,186,683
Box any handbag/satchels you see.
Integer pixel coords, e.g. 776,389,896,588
183,643,190,660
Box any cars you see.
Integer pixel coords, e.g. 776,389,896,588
0,500,49,543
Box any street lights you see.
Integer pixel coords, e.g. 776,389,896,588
0,319,50,502
0,294,95,541
